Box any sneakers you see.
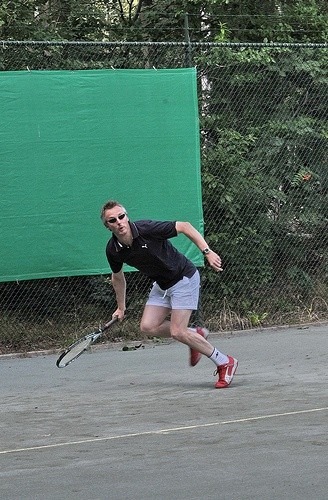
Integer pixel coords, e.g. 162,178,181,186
213,354,238,389
189,326,209,367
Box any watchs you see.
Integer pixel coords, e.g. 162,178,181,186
202,247,211,255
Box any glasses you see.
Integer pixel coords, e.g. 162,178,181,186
104,212,126,226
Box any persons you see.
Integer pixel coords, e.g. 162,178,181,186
100,200,239,389
